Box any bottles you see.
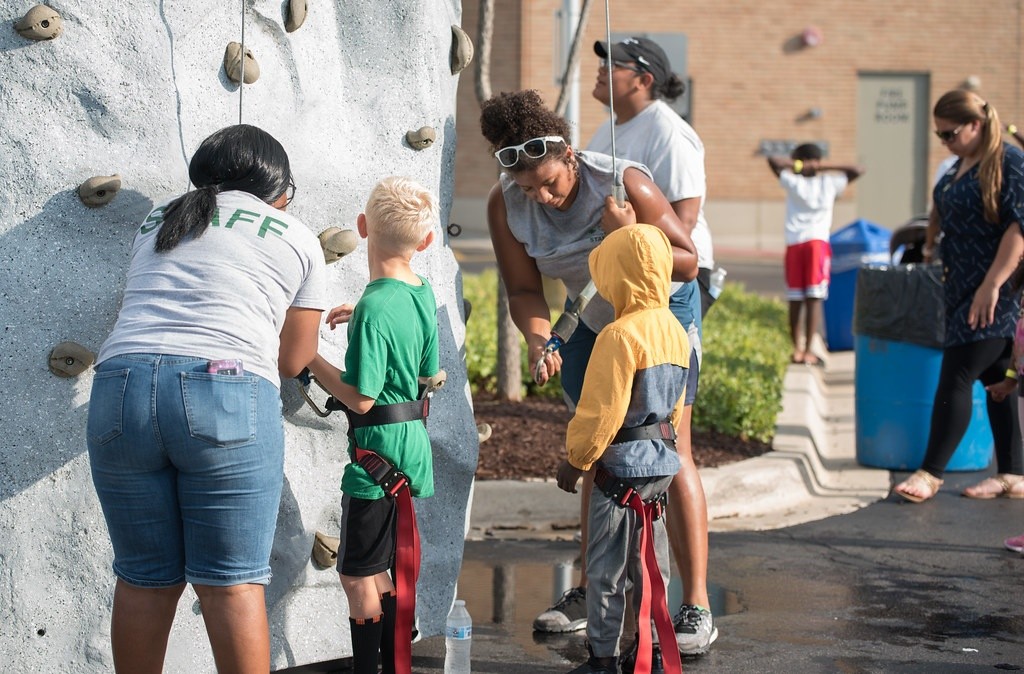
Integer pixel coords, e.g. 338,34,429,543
443,600,472,674
708,267,727,300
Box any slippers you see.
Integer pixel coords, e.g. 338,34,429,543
791,353,824,366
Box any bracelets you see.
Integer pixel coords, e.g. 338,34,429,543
794,159,804,174
922,243,934,256
1006,368,1018,380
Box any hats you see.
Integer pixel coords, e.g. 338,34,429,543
594,37,670,84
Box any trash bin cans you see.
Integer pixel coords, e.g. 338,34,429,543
823,220,893,351
856,261,993,471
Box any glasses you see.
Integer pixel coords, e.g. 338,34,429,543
495,136,567,167
599,58,640,72
286,183,297,200
935,122,964,143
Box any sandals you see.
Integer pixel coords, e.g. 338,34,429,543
962,474,1024,499
895,469,944,502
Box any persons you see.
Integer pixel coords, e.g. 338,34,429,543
479,37,720,655
893,86,1024,554
306,176,440,674
767,143,866,365
86,124,326,674
556,223,691,674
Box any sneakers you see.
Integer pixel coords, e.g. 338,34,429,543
621,647,663,674
672,603,718,656
532,584,587,632
567,656,622,674
1004,534,1024,552
